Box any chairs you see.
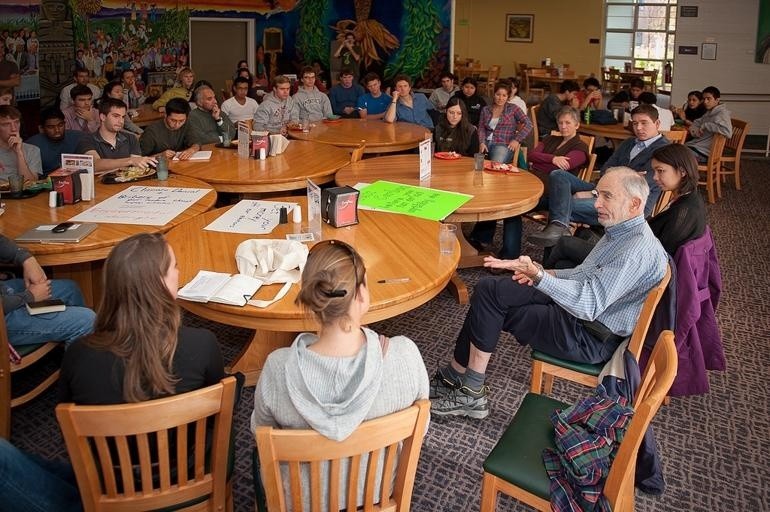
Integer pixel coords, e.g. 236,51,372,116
56,376,235,511
693,133,727,203
646,226,727,399
530,266,672,399
437,59,691,215
3,340,59,437
256,399,430,510
719,117,747,191
481,330,676,510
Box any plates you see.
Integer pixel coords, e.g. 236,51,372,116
483,161,514,173
102,166,156,184
231,139,252,145
321,118,342,124
436,152,462,161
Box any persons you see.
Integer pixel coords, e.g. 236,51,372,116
335,33,361,85
251,239,430,512
0,436,85,512
0,26,39,71
0,60,734,244
542,143,706,270
429,166,669,420
75,22,189,81
0,234,97,353
60,232,223,494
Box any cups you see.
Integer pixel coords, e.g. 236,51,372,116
220,132,232,150
7,174,22,194
474,153,487,172
436,223,458,254
157,156,169,180
302,119,310,133
544,56,565,79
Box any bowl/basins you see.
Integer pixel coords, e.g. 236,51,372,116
328,115,341,120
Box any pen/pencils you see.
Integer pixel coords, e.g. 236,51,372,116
377,278,411,283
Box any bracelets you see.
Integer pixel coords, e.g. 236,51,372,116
530,265,544,282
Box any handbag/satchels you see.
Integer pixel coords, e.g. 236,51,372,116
235,237,309,284
581,109,616,126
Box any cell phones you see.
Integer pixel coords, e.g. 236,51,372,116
53,223,70,232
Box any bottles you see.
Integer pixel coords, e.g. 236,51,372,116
279,207,289,221
293,205,302,223
254,149,260,159
48,190,64,209
260,148,265,160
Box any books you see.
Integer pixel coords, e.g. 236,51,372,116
27,300,66,316
177,268,263,307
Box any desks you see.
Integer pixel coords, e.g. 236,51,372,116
162,196,462,387
2,172,217,313
127,105,164,128
284,116,435,158
157,140,353,203
334,154,544,306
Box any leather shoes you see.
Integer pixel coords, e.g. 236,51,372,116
527,223,573,248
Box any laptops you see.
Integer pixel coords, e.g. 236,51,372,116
14,224,96,243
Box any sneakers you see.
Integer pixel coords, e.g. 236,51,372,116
429,366,490,419
488,254,519,276
466,240,495,254
